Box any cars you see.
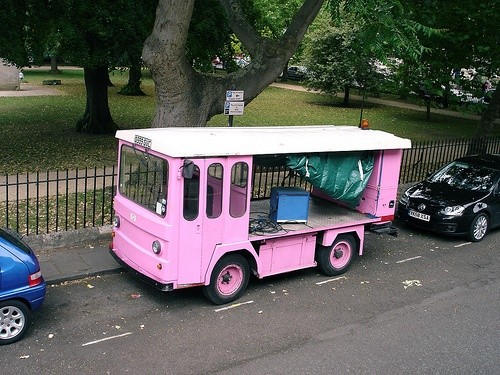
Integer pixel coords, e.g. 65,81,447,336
286,65,309,80
395,153,500,243
0,226,46,346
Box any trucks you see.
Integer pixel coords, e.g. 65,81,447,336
108,118,412,305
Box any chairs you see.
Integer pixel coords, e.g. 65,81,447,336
207,185,213,217
